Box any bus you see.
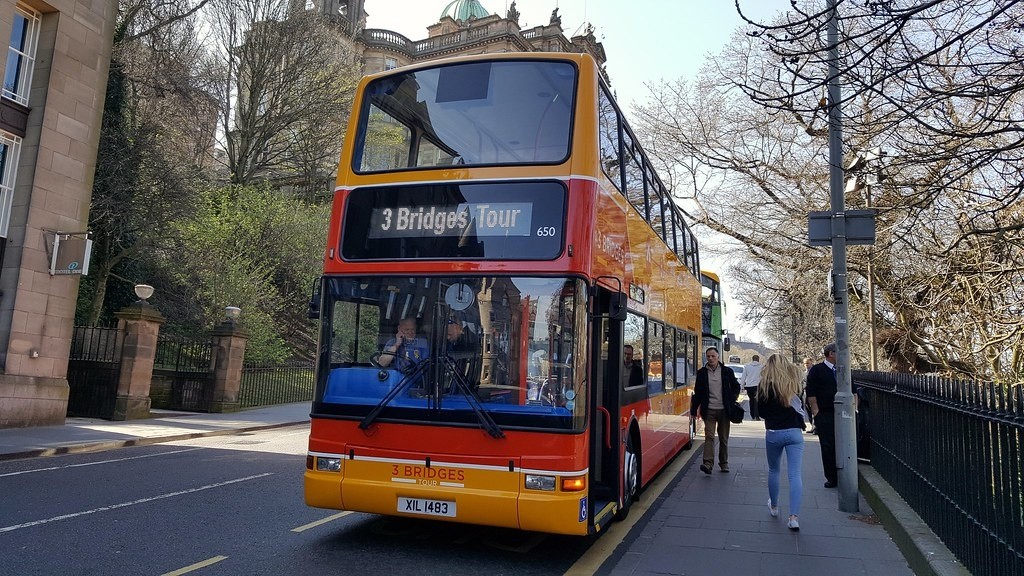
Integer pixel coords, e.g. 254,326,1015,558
305,53,740,535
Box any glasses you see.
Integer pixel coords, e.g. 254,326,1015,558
401,328,416,334
449,320,459,326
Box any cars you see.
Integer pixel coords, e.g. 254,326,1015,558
724,365,747,392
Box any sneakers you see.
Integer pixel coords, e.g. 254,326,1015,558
788,519,799,529
768,498,778,517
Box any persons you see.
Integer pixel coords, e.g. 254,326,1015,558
800,342,858,488
740,355,766,421
690,348,740,474
623,344,644,389
379,316,484,396
756,353,803,530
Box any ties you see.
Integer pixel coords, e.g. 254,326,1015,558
833,367,836,380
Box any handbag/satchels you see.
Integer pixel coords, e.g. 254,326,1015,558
728,402,745,423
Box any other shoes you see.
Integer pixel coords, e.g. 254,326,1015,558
752,417,761,421
721,467,730,473
700,464,712,474
824,480,838,488
806,429,817,435
